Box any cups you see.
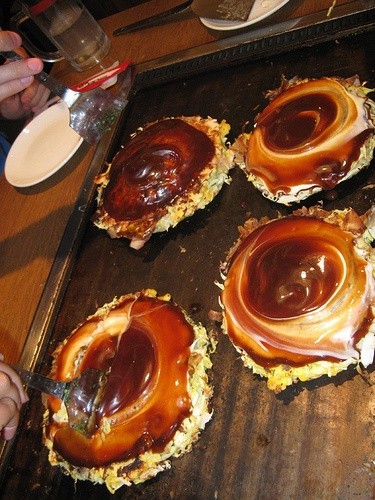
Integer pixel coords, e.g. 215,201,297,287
6,0,112,73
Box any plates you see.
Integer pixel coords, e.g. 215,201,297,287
4,101,85,188
198,0,289,31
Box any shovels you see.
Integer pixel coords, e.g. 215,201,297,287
113,0,256,37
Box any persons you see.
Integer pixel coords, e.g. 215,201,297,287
0,354,29,443
0,30,52,173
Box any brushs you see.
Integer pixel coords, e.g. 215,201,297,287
8,363,108,438
0,49,128,147
